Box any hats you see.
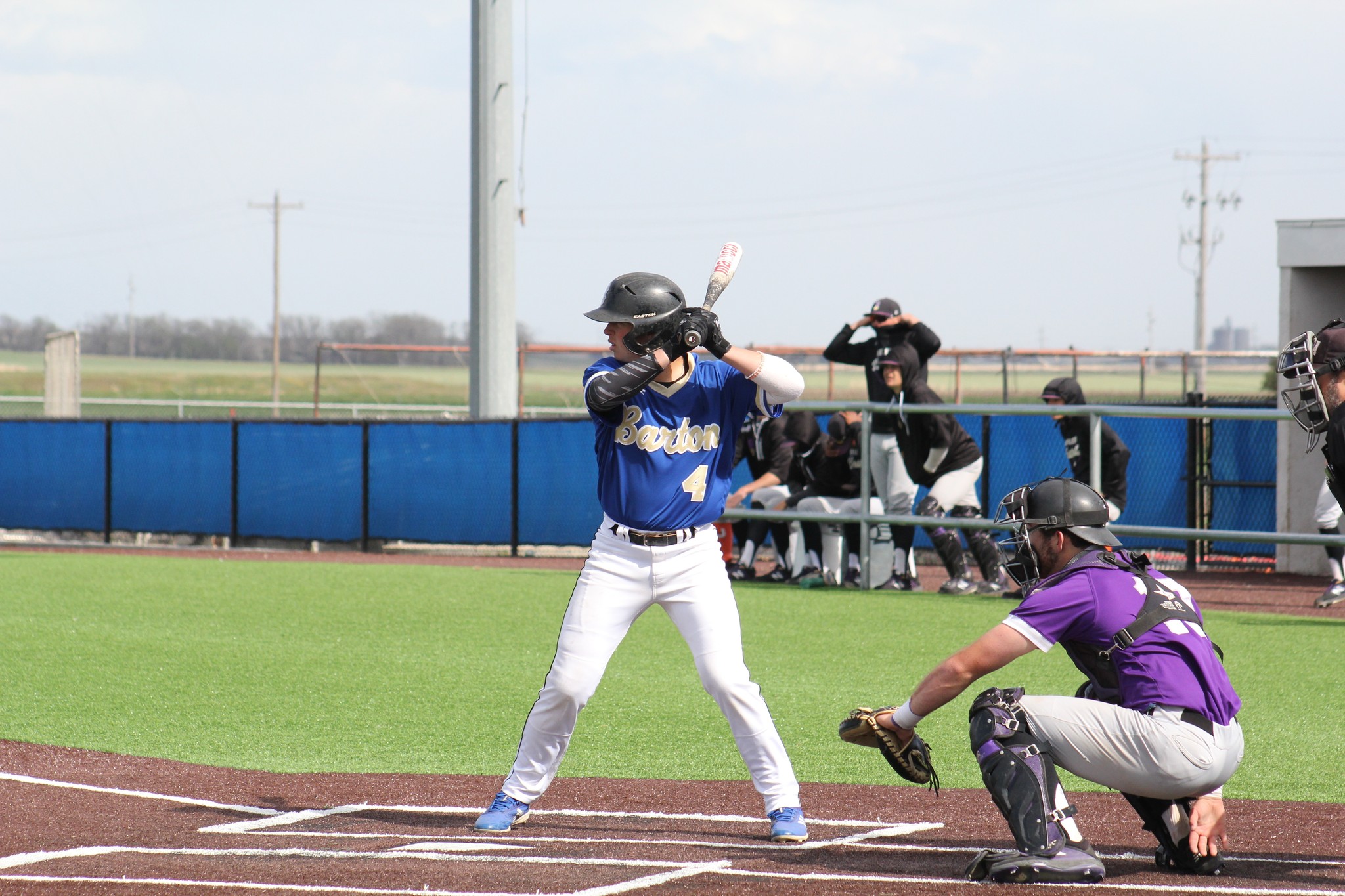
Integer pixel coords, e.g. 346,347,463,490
863,298,901,318
1042,390,1060,399
875,351,899,366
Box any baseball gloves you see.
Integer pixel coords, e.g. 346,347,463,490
839,705,932,785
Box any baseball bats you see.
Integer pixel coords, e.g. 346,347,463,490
684,241,743,347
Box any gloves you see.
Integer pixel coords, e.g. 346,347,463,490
661,308,731,362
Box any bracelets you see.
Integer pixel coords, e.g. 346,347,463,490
891,696,926,731
1202,784,1224,800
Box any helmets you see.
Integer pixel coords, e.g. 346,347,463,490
1276,320,1345,433
993,475,1123,599
582,272,686,355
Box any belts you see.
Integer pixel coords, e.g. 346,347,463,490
1142,706,1213,737
610,524,695,546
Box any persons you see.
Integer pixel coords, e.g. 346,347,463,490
837,476,1245,883
1276,318,1345,513
1036,377,1131,553
822,298,942,590
1313,473,1345,609
472,270,810,842
876,341,1009,598
724,410,884,590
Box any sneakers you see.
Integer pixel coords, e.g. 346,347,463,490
752,564,791,583
1154,842,1222,875
787,562,861,589
725,563,754,581
937,577,977,595
965,838,1104,885
873,570,920,591
1313,581,1345,610
767,807,810,843
474,789,530,832
974,576,1011,596
